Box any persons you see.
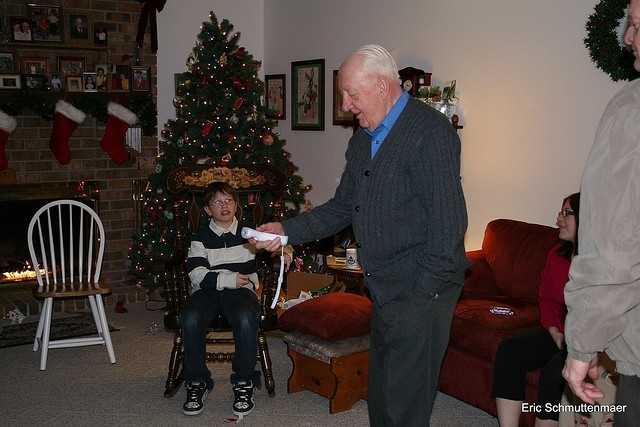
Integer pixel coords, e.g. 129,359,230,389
490,192,577,427
251,43,473,425
562,0,639,427
179,181,259,414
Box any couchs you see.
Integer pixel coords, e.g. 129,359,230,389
434,217,619,427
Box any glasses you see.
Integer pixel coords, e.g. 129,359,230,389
206,197,237,208
559,210,579,217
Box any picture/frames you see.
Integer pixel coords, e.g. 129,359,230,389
69,13,88,40
173,71,190,120
290,58,326,132
26,3,65,44
264,73,286,121
9,15,33,43
92,26,108,48
0,52,150,93
332,70,358,128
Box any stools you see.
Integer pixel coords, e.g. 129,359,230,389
279,292,375,410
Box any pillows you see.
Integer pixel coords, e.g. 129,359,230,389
278,291,375,341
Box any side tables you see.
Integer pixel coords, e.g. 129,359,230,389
319,266,370,300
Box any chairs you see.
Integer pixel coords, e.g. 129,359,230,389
26,199,117,372
159,251,276,399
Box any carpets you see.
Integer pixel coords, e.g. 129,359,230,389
0,312,123,350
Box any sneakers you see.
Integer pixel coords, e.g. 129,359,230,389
182,378,209,416
232,380,256,417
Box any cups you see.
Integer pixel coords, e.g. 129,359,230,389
345,248,360,269
